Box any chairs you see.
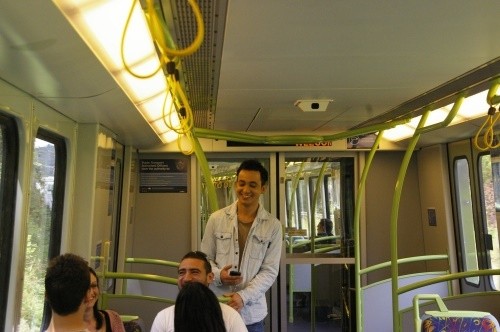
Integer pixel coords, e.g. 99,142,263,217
284,226,310,249
420,309,500,332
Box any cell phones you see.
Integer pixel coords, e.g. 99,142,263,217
230,271,241,276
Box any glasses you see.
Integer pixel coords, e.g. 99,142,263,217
185,251,210,264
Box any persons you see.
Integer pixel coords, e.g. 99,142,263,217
315,219,336,244
150,251,248,332
43,253,126,332
200,160,283,332
